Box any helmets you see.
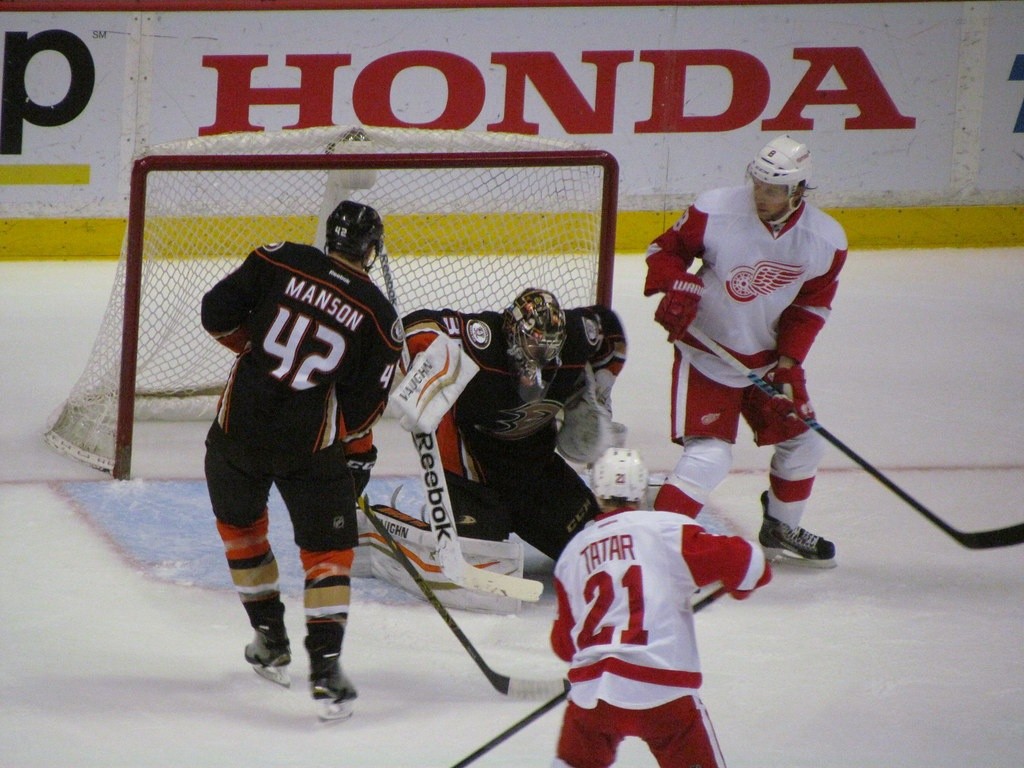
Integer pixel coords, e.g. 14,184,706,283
326,200,384,257
594,447,646,501
502,288,566,385
748,135,809,196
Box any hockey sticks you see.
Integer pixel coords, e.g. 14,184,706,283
686,324,1024,551
378,238,545,603
356,495,572,702
451,587,727,768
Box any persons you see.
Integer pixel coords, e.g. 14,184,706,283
644,132,849,568
202,199,406,722
550,448,771,768
352,287,628,609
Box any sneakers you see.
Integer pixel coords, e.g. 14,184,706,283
242,602,291,686
304,631,358,720
757,490,837,570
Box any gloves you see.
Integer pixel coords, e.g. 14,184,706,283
770,366,809,425
654,273,704,343
732,564,773,601
345,444,377,499
